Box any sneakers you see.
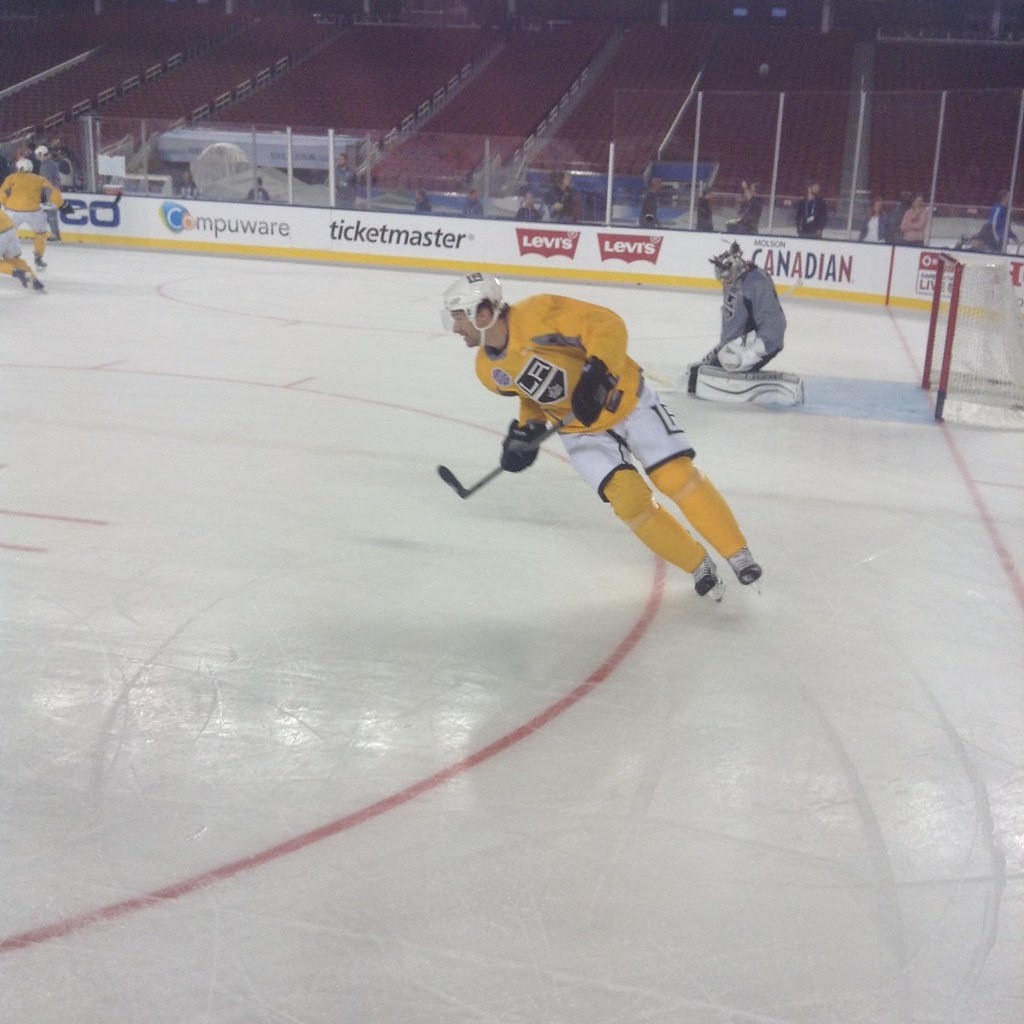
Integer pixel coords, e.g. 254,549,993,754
13,268,27,289
690,553,717,596
34,254,48,266
727,545,761,585
33,281,45,290
46,233,61,241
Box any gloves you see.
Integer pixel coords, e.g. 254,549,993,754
571,356,617,425
500,418,547,473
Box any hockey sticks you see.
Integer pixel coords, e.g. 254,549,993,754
439,410,581,499
44,192,122,212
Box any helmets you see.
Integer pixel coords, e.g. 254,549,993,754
34,146,48,160
708,238,743,268
15,158,33,171
443,272,503,323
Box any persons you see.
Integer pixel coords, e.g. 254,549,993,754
0,135,75,294
247,150,1023,256
683,239,808,409
445,270,764,606
